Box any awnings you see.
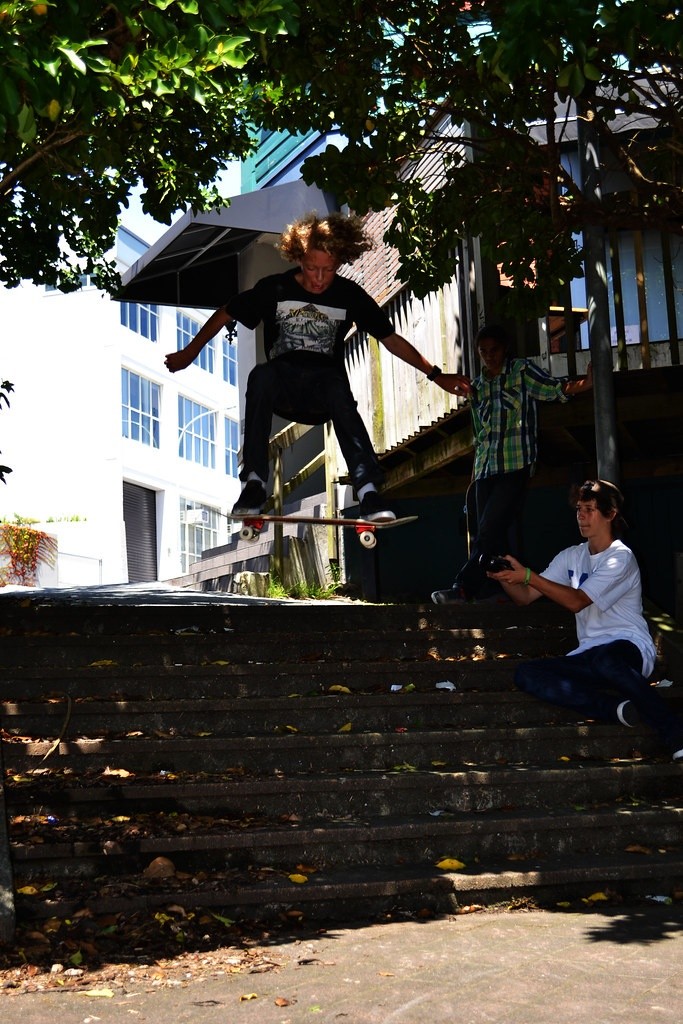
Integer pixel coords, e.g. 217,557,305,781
110,178,341,310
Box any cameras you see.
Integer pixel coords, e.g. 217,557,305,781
479,553,512,574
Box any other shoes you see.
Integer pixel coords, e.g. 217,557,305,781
431,590,465,604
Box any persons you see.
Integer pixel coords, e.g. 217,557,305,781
488,479,683,760
431,320,594,603
164,215,473,521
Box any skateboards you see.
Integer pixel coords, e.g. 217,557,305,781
197,497,421,551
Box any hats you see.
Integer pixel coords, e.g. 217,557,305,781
586,480,629,529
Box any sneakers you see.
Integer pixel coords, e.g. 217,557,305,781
360,491,396,521
672,745,683,762
233,479,268,514
614,698,641,728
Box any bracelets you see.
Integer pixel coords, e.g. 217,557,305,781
427,365,441,381
524,568,531,585
